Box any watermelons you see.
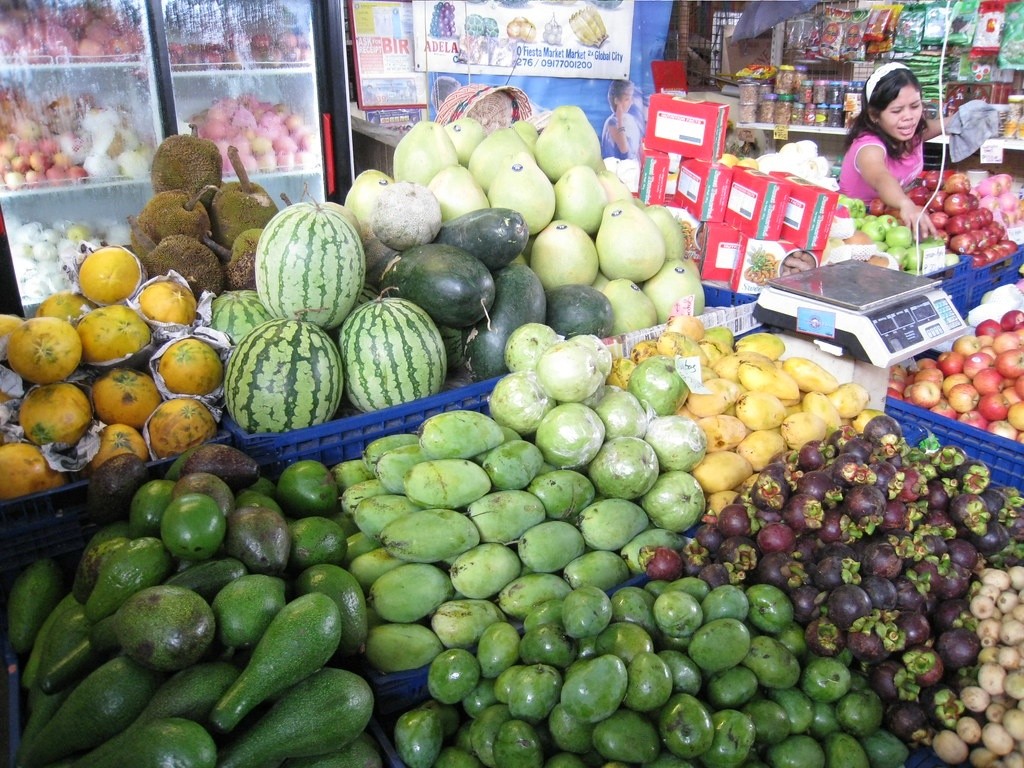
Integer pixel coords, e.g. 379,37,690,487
209,200,466,435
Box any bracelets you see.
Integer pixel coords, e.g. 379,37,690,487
616,127,625,133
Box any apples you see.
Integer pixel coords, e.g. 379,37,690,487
835,169,1024,275
888,309,1024,446
0,0,317,303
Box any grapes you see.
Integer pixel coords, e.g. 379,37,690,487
430,2,457,38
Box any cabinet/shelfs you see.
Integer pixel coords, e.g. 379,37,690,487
735,0,1024,196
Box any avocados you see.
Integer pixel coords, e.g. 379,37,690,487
0,443,392,768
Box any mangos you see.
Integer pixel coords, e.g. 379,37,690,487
394,576,912,768
323,315,886,676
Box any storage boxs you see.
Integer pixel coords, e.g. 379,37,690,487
0,428,232,580
699,224,740,281
922,255,974,320
646,93,730,162
665,151,685,205
221,377,506,483
769,171,839,250
725,165,793,241
676,157,733,224
971,247,1022,314
638,137,670,206
883,393,1024,497
730,233,824,293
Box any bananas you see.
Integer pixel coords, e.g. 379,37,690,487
569,5,609,48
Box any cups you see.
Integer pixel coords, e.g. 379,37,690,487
967,170,989,187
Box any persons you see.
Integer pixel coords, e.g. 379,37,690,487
601,80,639,159
838,62,952,241
431,77,461,114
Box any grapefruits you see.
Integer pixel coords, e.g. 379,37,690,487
349,102,706,336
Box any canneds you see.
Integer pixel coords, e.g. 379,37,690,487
738,63,868,129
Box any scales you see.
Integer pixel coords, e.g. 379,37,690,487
751,258,968,368
